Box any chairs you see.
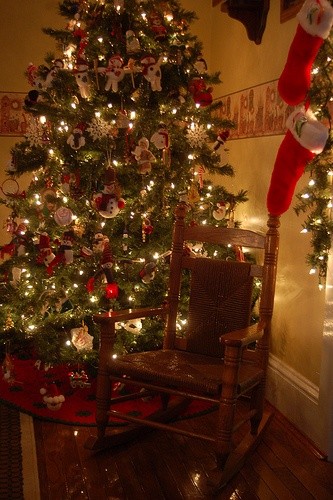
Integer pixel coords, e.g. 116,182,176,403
82,201,281,491
